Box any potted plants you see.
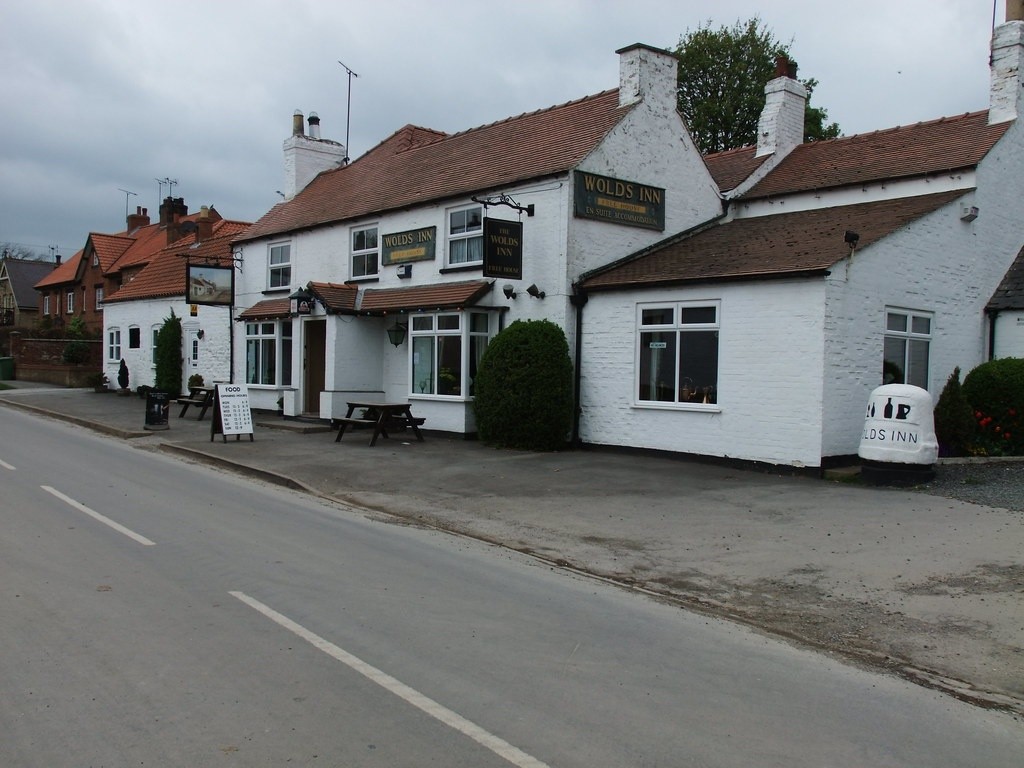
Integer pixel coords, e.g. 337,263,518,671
116,358,130,396
86,371,110,393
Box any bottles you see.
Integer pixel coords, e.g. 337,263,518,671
681,385,697,401
702,385,711,403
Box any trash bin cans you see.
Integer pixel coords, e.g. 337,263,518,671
144,392,169,430
0,356,17,380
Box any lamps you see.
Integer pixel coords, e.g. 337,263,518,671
842,229,864,266
526,283,546,300
287,287,315,314
503,285,517,300
196,329,204,340
387,318,408,349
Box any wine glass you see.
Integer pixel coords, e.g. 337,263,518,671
419,381,426,393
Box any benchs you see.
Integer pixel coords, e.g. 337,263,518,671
331,415,426,446
177,399,208,421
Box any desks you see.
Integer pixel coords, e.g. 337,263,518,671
335,402,425,447
179,386,216,421
